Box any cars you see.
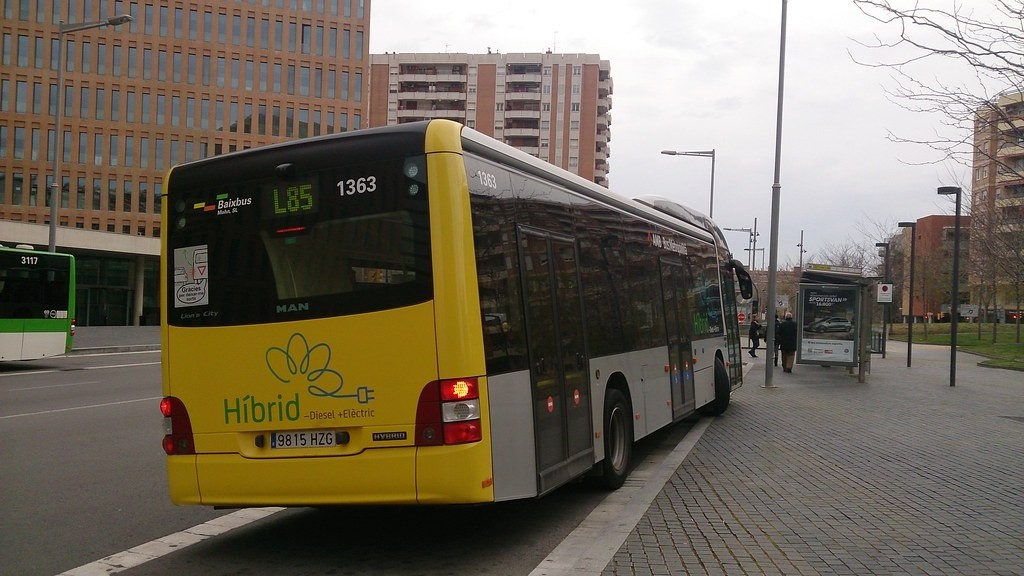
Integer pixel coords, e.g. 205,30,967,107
807,317,853,334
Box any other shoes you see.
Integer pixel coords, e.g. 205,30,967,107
748,351,758,358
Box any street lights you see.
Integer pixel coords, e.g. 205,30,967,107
897,221,916,367
936,185,963,387
743,248,766,271
659,148,717,220
875,242,889,358
46,13,135,283
720,226,752,272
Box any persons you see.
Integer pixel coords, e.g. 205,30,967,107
764,315,780,366
776,313,797,372
749,316,761,357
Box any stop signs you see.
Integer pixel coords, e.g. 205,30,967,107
738,313,746,322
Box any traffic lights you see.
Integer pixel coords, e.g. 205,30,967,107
1020,311,1022,319
941,312,945,319
938,312,942,320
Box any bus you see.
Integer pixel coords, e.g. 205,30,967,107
158,118,753,507
0,244,77,362
